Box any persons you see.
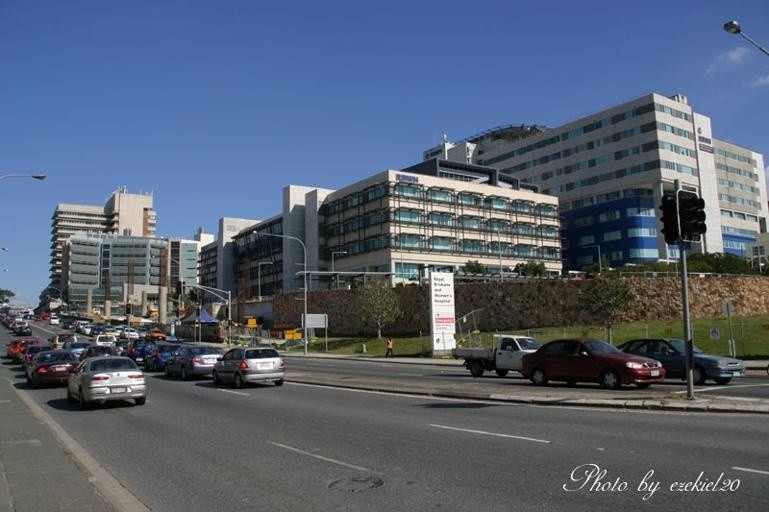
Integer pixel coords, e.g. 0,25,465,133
385,334,393,356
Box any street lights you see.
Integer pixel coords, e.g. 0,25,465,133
723,20,768,58
253,230,309,356
0,172,46,183
0,269,10,275
166,256,184,304
493,227,504,283
0,246,8,252
331,250,347,271
257,260,274,300
179,282,232,348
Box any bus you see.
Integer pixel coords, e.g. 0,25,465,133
568,270,587,280
492,271,519,280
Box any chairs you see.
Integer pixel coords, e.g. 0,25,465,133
94,363,130,371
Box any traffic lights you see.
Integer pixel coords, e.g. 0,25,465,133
658,191,679,244
677,189,706,243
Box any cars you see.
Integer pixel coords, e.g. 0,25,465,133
165,344,221,381
613,337,747,385
398,279,418,286
210,347,286,387
146,329,166,342
69,355,148,409
522,337,666,389
136,328,147,337
24,349,79,385
0,303,139,363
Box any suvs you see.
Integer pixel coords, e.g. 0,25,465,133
144,342,180,371
126,339,156,365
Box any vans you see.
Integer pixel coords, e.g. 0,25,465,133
144,309,159,321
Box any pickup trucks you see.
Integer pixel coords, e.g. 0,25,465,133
452,333,541,379
